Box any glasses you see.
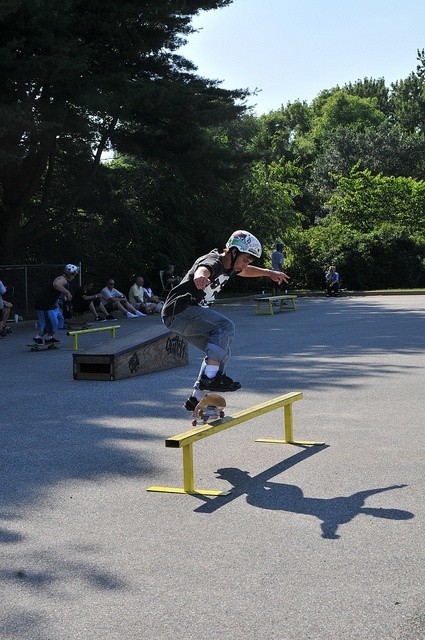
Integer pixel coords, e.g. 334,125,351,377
110,283,114,285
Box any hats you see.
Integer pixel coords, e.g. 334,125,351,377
276,243,284,249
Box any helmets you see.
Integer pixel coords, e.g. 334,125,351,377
225,230,262,259
64,264,78,276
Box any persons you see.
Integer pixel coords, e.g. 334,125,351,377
3,300,13,333
0,281,7,337
162,263,180,290
33,264,79,344
128,276,156,314
77,279,118,322
272,242,287,296
160,230,290,412
143,276,165,312
326,266,341,297
100,279,148,319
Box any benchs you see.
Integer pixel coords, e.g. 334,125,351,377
253,295,298,315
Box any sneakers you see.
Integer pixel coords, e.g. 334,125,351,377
0,324,12,337
95,315,106,322
199,370,241,392
105,314,117,321
45,337,61,345
136,313,146,317
128,315,138,319
33,336,44,345
183,394,224,418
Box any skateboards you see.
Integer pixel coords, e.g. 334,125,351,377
191,394,226,426
26,341,60,351
65,322,91,331
274,301,289,307
